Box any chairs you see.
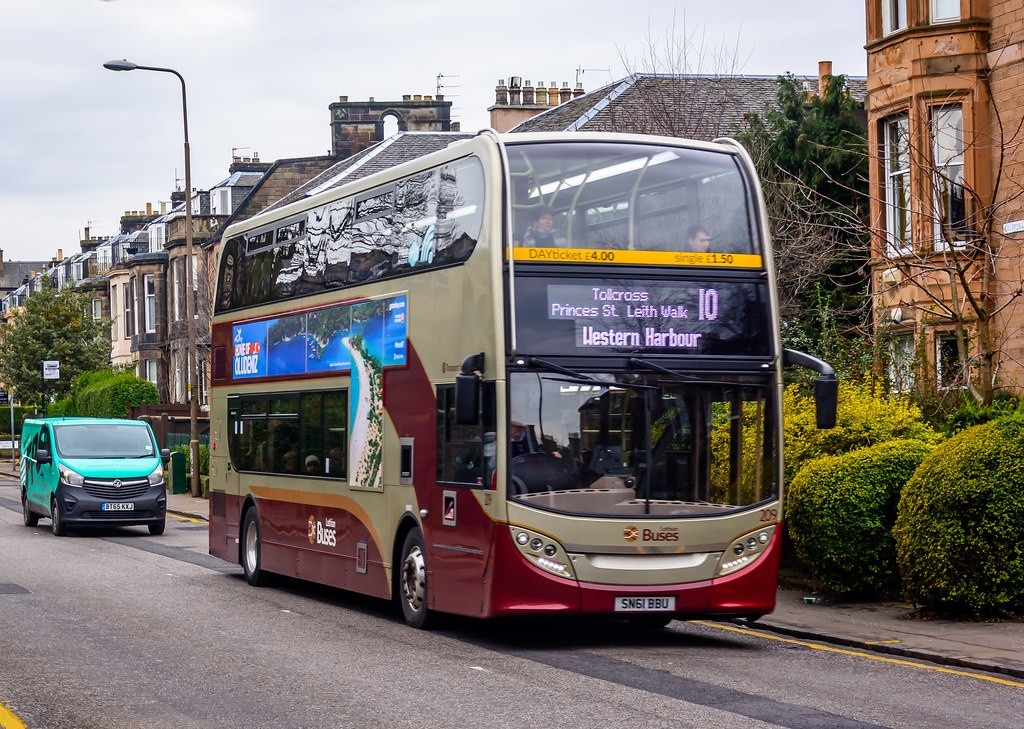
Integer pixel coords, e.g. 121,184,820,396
510,452,580,493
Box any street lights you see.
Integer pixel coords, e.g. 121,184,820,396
104,58,201,499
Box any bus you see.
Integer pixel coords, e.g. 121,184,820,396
205,128,842,631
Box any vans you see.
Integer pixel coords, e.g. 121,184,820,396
18,415,171,538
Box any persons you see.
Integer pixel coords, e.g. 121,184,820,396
681,223,712,253
284,448,346,477
487,409,562,472
521,204,565,247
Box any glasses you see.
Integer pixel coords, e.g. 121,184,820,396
692,237,712,243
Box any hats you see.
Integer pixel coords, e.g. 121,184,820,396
305,455,320,466
